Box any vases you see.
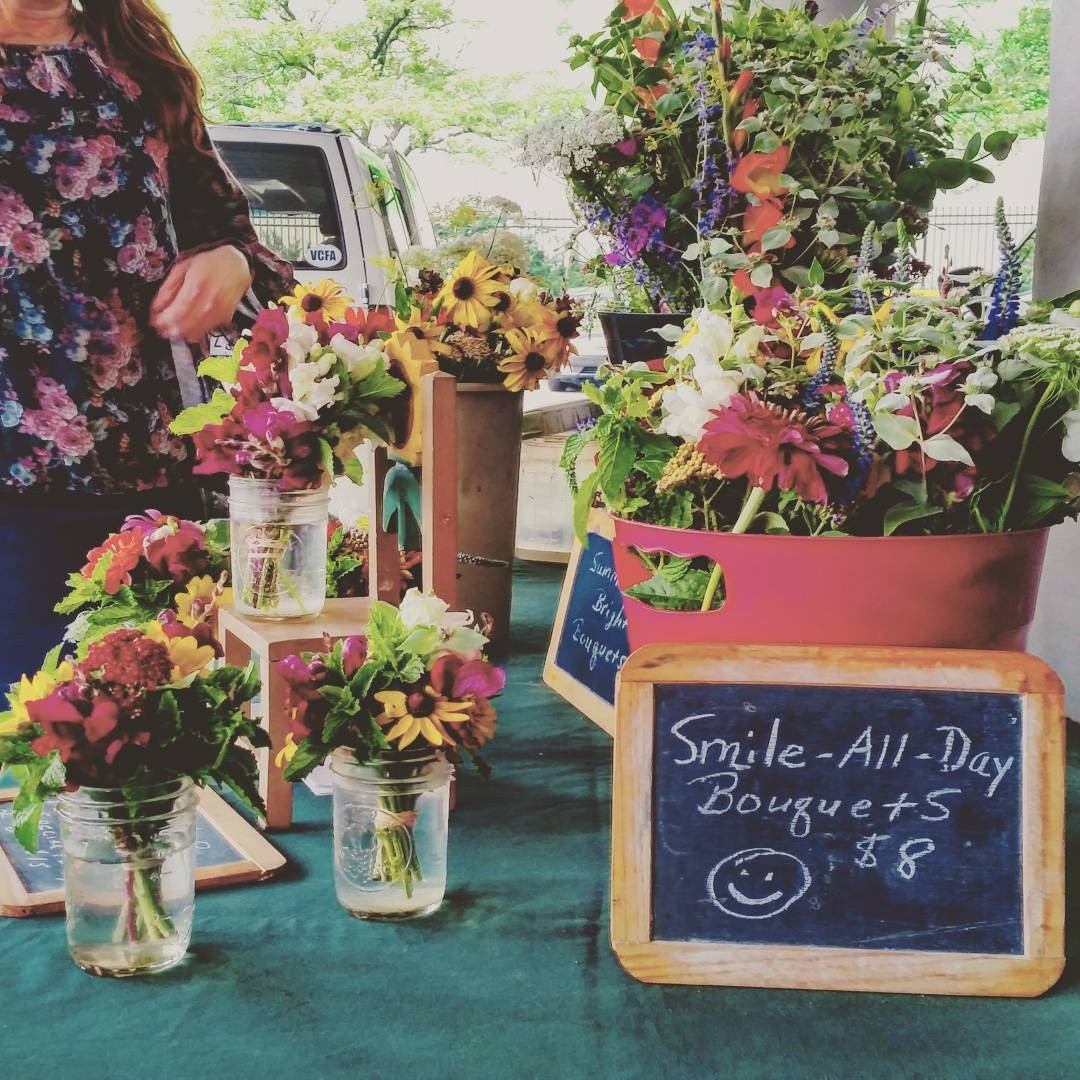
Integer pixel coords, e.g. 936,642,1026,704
453,380,524,649
54,774,200,975
599,310,694,369
335,742,454,918
231,470,330,614
609,515,1051,653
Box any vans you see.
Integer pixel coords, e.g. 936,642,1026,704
209,122,441,317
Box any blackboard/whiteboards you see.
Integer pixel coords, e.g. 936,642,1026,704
611,644,1066,996
0,778,288,918
542,507,630,739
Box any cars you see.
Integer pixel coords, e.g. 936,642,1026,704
547,333,611,391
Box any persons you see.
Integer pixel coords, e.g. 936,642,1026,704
0,0,300,717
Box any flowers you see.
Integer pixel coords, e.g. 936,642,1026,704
0,509,266,942
541,0,1079,611
169,276,407,604
277,589,505,892
376,188,579,390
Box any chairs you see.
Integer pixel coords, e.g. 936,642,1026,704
219,372,455,831
